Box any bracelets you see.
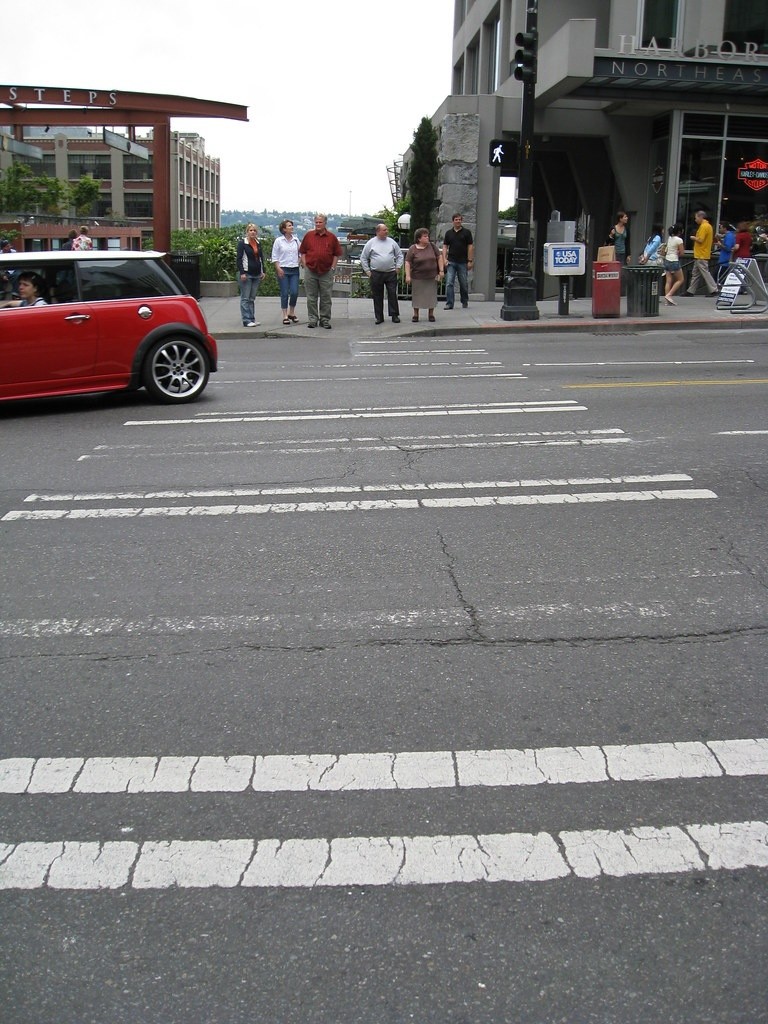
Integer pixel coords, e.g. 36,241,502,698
467,259,473,263
439,270,444,272
331,266,336,272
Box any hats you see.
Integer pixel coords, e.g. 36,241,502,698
68,229,79,237
1,240,9,250
80,226,89,233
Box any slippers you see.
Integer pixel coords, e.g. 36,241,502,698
283,319,290,324
288,315,299,323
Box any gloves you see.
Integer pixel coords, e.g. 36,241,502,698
260,273,265,280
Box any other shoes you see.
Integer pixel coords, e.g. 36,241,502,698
307,323,317,328
375,320,384,324
429,315,435,322
705,291,720,297
412,315,418,322
392,316,400,323
244,321,261,327
319,320,331,329
665,294,677,305
679,291,694,297
444,304,453,310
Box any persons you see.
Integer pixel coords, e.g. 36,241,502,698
0,240,25,294
236,222,266,327
298,214,342,329
271,219,303,325
0,271,49,309
639,222,667,303
442,213,474,311
360,223,404,324
713,220,753,295
664,223,685,307
61,225,93,251
405,228,444,322
603,211,632,298
679,210,720,298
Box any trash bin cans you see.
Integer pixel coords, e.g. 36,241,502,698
623,265,664,317
168,250,202,300
592,261,621,318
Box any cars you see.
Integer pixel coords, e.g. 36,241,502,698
0,247,218,415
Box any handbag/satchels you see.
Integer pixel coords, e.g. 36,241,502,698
262,267,266,273
657,243,667,256
437,265,447,274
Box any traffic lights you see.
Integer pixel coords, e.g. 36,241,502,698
489,139,519,169
514,32,535,81
